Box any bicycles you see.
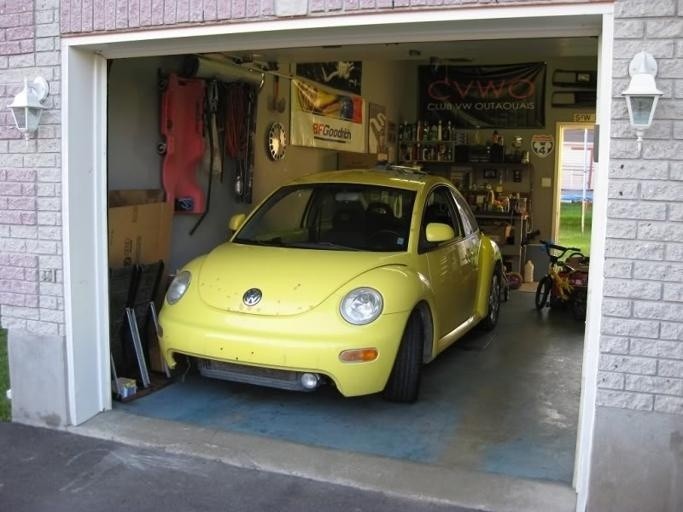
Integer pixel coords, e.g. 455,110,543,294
536,239,588,320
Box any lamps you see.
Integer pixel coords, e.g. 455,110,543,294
6,76,50,141
621,52,663,154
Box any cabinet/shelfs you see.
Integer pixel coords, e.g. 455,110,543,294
397,140,532,273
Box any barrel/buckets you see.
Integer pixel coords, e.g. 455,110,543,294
524,260,534,282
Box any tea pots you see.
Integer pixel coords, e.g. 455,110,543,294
514,198,527,214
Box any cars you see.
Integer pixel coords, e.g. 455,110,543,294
157,168,503,402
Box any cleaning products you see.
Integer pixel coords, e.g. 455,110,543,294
437,120,442,141
417,119,422,142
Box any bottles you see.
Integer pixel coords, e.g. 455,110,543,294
488,129,502,162
416,120,452,161
510,137,524,158
